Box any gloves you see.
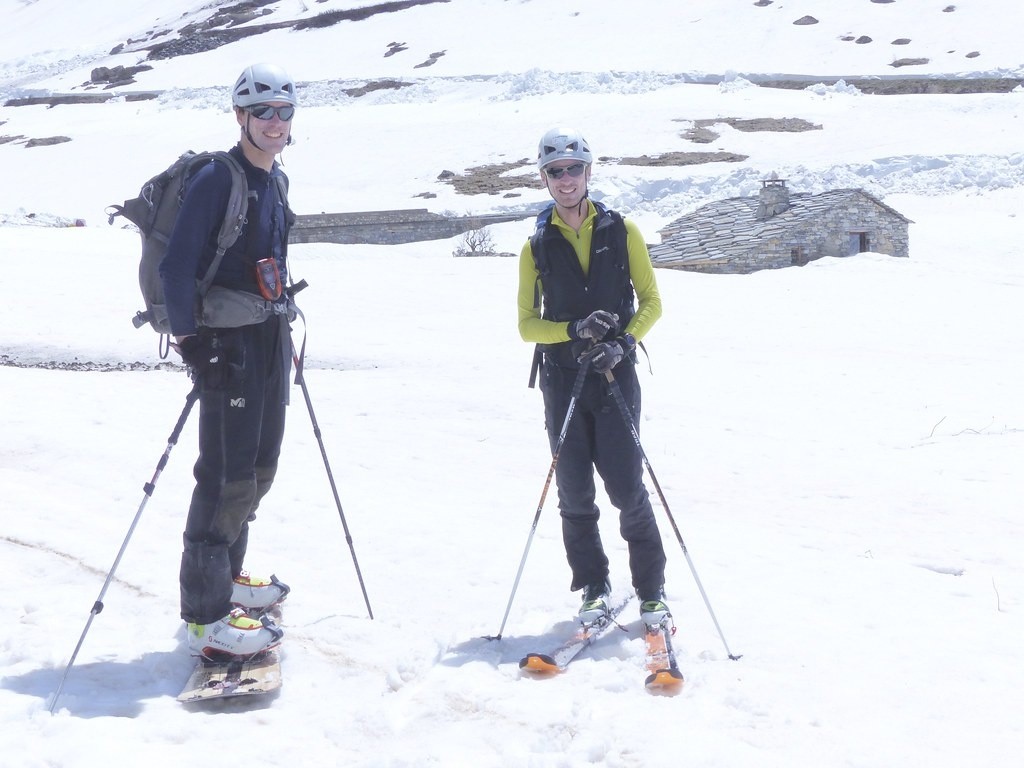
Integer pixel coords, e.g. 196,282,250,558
288,297,299,322
178,336,225,390
568,310,620,341
577,336,630,373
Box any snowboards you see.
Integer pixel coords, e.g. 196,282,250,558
172,595,286,705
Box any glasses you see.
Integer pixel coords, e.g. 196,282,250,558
239,106,295,121
543,164,587,178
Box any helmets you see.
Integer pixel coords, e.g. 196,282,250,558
537,127,593,169
232,63,297,107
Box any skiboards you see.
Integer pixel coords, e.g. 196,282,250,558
517,583,684,690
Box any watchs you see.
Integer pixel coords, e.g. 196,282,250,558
620,332,635,350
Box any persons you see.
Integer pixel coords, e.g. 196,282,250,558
158,62,299,656
517,126,669,623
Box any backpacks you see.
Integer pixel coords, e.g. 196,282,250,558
104,158,297,335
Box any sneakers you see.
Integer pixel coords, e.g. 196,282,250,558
187,609,283,665
637,586,672,635
579,575,611,629
231,571,290,614
